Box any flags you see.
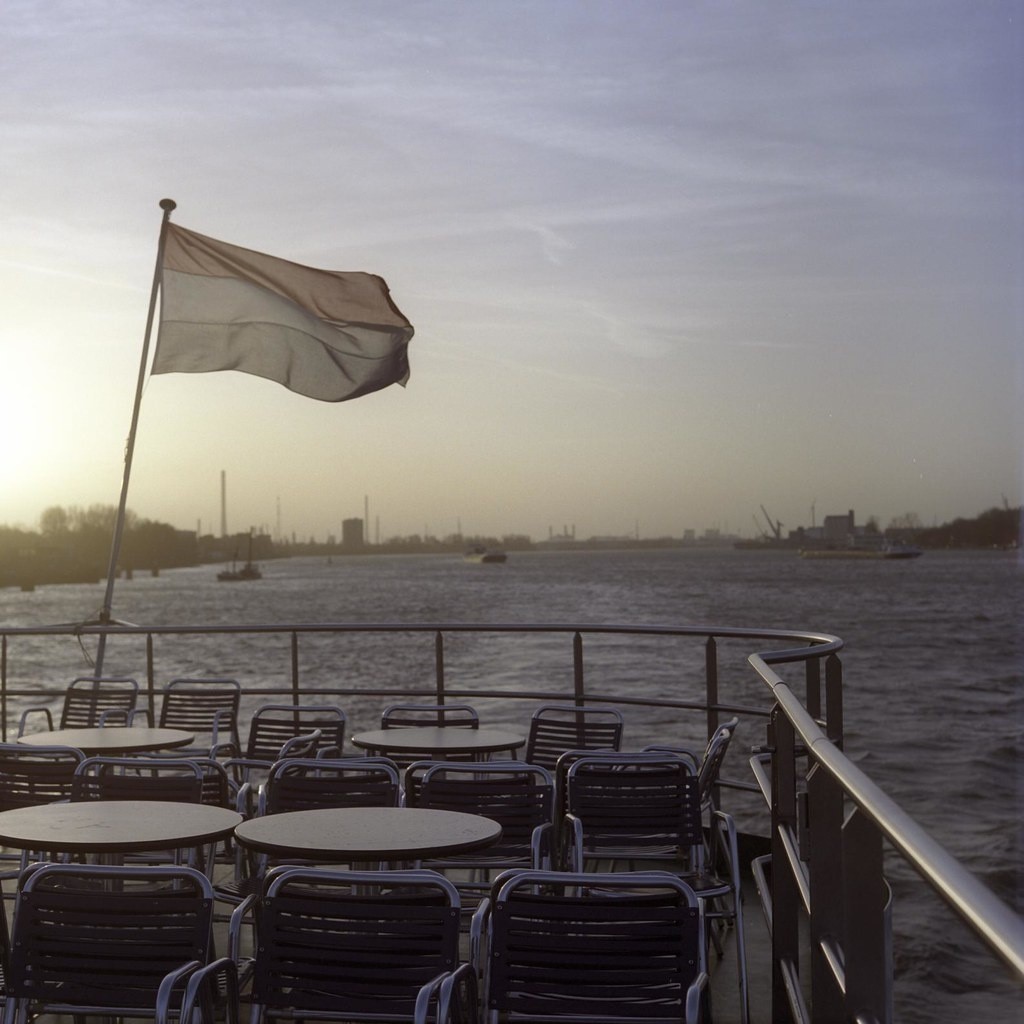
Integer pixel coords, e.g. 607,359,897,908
152,223,414,405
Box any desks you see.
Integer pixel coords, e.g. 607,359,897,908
352,728,526,877
17,727,195,865
234,807,501,898
0,800,243,1024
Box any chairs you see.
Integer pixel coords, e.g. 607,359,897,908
0,674,754,1023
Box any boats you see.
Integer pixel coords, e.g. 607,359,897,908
217,526,263,582
479,550,509,564
796,546,924,561
